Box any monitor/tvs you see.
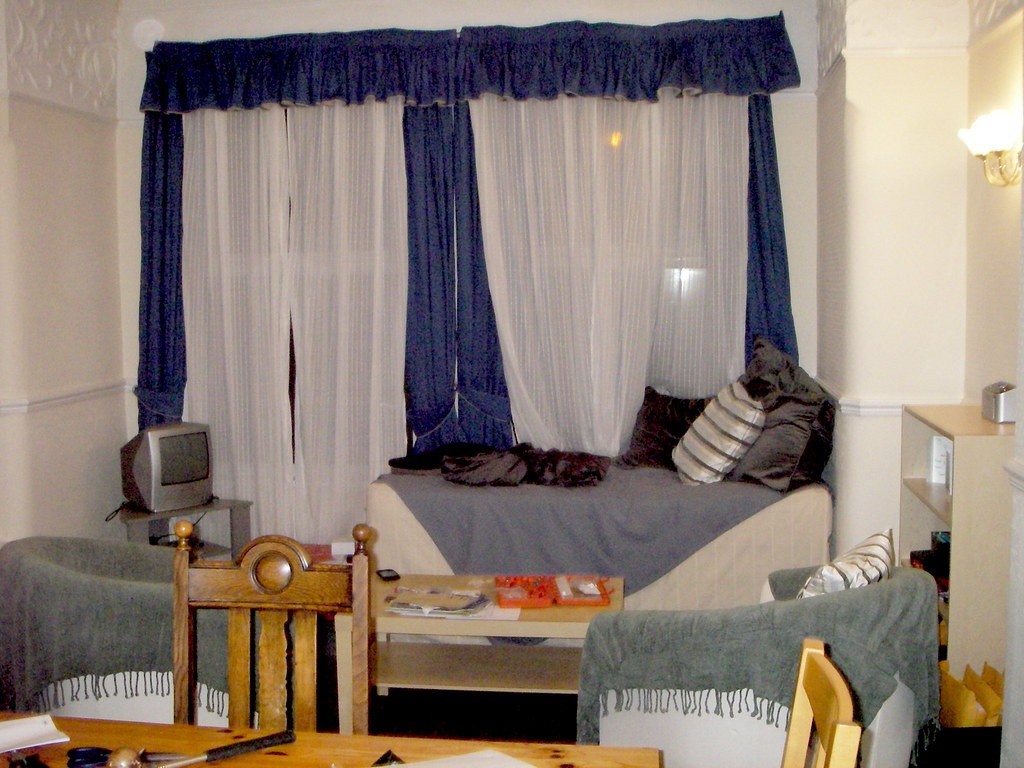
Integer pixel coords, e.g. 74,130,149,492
121,422,213,513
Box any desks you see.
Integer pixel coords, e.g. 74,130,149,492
119,499,255,560
374,572,626,698
0,711,663,768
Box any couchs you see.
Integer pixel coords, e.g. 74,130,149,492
367,460,832,648
0,535,296,729
578,563,940,768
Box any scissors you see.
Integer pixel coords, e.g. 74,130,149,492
66,746,186,768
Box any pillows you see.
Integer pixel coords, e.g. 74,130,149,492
672,379,766,487
796,528,896,601
722,337,838,493
616,386,717,474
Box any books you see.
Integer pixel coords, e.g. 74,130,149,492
382,584,521,621
0,713,71,754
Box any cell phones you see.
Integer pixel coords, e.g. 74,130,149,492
376,570,401,581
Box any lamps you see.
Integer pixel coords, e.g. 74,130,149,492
958,102,1024,188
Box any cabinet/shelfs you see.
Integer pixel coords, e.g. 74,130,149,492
898,406,1017,729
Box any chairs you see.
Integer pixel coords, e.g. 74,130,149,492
173,520,370,736
780,639,861,768
